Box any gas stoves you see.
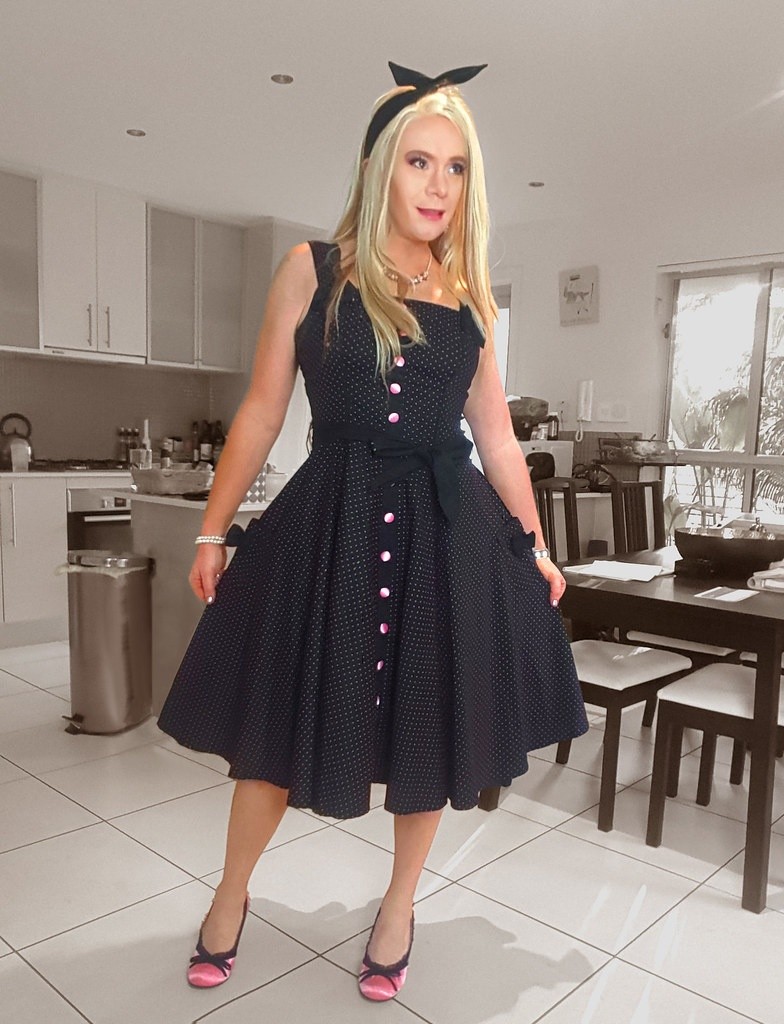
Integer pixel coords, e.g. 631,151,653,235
33,458,129,472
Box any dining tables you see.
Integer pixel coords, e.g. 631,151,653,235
550,529,784,914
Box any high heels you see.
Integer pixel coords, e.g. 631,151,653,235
185,883,250,987
358,893,417,1001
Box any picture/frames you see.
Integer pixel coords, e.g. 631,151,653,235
558,264,601,327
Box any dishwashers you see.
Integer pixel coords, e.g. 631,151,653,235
66,486,135,557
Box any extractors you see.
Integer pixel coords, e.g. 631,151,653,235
42,346,146,367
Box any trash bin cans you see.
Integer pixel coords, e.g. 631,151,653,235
60,549,155,734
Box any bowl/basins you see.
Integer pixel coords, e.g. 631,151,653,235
620,440,663,458
676,526,784,572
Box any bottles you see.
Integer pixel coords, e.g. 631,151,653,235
118,427,142,464
191,419,225,471
142,419,152,449
547,411,559,440
530,423,548,441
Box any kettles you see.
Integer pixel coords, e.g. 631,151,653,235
1,413,35,471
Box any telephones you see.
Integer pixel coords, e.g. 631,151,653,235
577,380,593,421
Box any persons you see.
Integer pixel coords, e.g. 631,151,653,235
185,82,567,1003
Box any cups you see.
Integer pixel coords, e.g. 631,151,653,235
264,473,288,501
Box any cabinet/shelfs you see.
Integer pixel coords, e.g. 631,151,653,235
0,459,226,645
0,164,253,376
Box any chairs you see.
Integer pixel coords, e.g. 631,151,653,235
610,478,749,731
476,476,694,837
645,657,784,855
